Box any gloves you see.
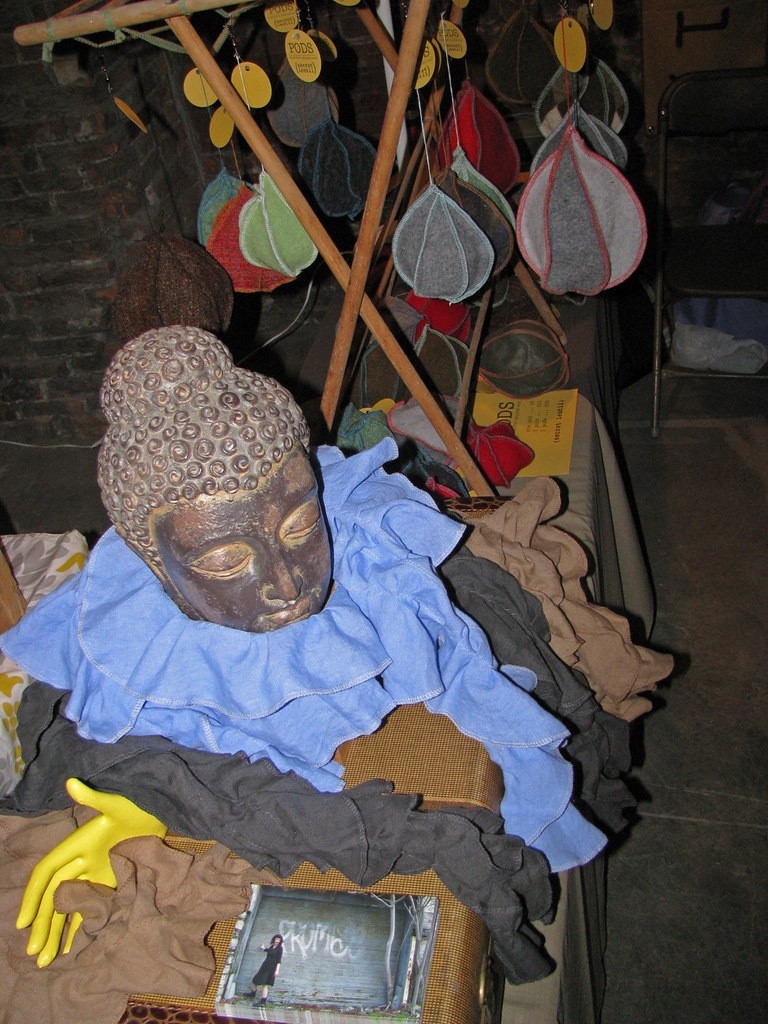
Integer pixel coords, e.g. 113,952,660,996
16,776,173,969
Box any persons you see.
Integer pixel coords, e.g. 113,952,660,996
96,326,334,632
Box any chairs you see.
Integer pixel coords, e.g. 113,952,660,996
651,69,768,439
